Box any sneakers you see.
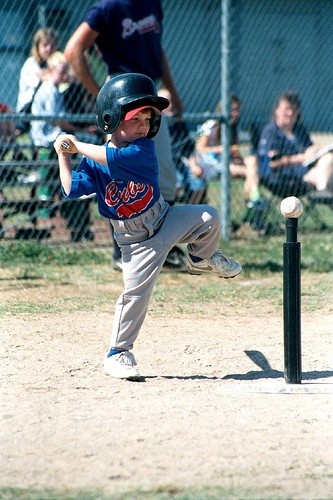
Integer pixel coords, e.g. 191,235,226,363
72,232,95,242
104,351,142,382
159,250,193,272
112,260,124,270
187,251,245,281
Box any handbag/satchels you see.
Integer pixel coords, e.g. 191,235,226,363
14,81,43,133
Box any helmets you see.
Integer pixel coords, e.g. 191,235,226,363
95,73,172,140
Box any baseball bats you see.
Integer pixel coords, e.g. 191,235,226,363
57,135,74,152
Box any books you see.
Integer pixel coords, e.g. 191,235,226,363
302,144,332,168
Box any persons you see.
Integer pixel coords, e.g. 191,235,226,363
14,27,65,219
64,0,184,273
156,86,209,206
257,92,332,203
195,94,263,201
55,71,241,380
29,50,69,232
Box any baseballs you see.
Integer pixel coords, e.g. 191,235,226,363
279,195,305,219
